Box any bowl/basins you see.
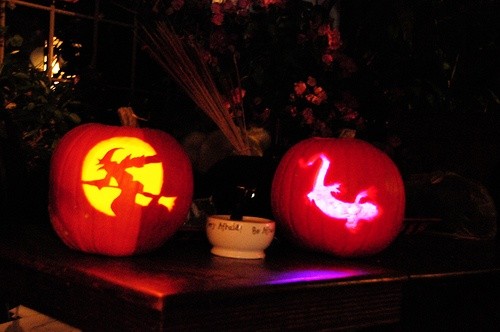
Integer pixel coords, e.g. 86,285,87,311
206,214,277,260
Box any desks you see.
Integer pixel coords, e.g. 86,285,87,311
1,246,500,332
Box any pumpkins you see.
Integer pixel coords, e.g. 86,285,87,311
270,128,405,257
47,106,195,256
